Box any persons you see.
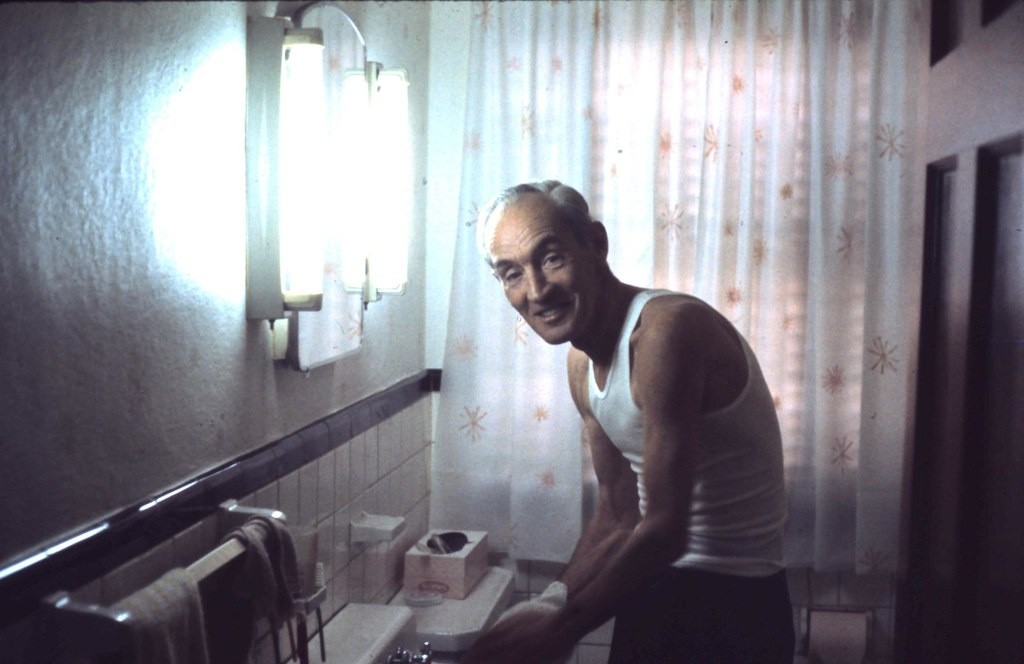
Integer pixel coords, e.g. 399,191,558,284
427,181,796,664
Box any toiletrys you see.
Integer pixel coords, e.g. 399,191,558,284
284,529,319,597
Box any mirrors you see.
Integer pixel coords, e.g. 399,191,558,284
289,1,367,374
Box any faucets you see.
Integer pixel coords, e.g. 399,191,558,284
397,640,433,664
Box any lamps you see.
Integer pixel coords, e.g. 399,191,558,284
374,66,415,298
280,25,329,313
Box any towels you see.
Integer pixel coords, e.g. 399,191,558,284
222,517,302,621
106,564,208,664
